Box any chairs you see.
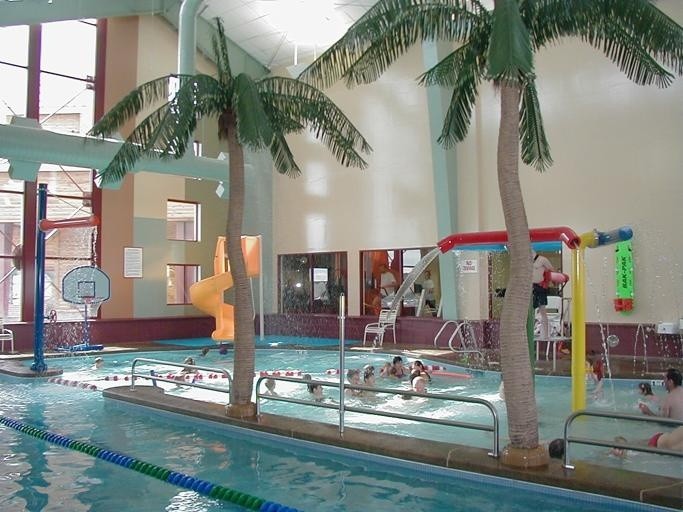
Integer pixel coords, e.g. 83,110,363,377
0,316,14,354
533,306,564,370
360,308,398,349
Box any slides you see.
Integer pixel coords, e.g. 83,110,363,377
191,237,262,341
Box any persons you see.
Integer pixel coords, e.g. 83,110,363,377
174,356,199,387
547,438,564,458
372,262,398,316
89,356,104,371
261,378,278,397
584,344,682,462
198,347,209,357
219,347,227,355
530,248,554,339
421,270,437,317
302,354,432,403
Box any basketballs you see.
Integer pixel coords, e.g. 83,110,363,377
219,347,228,354
